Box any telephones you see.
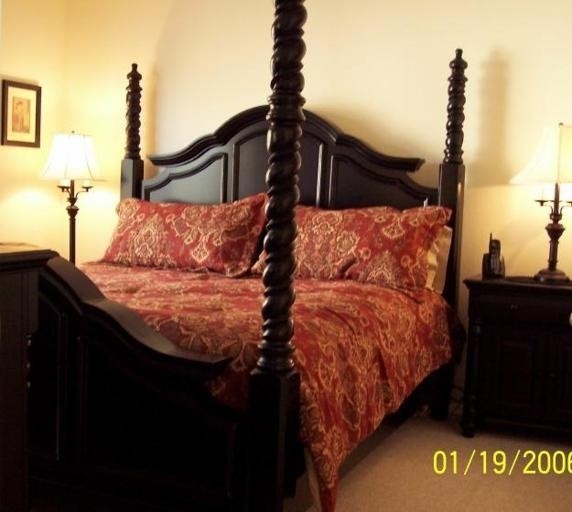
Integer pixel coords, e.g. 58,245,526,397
482,233,506,280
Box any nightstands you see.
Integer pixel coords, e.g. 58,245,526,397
459,274,572,438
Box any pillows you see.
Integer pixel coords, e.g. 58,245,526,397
248,206,452,304
82,193,269,279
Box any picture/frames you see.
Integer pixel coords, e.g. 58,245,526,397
2,80,41,148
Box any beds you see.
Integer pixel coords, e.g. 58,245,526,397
0,1,467,512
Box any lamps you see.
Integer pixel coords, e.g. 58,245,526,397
38,129,106,267
511,121,571,283
